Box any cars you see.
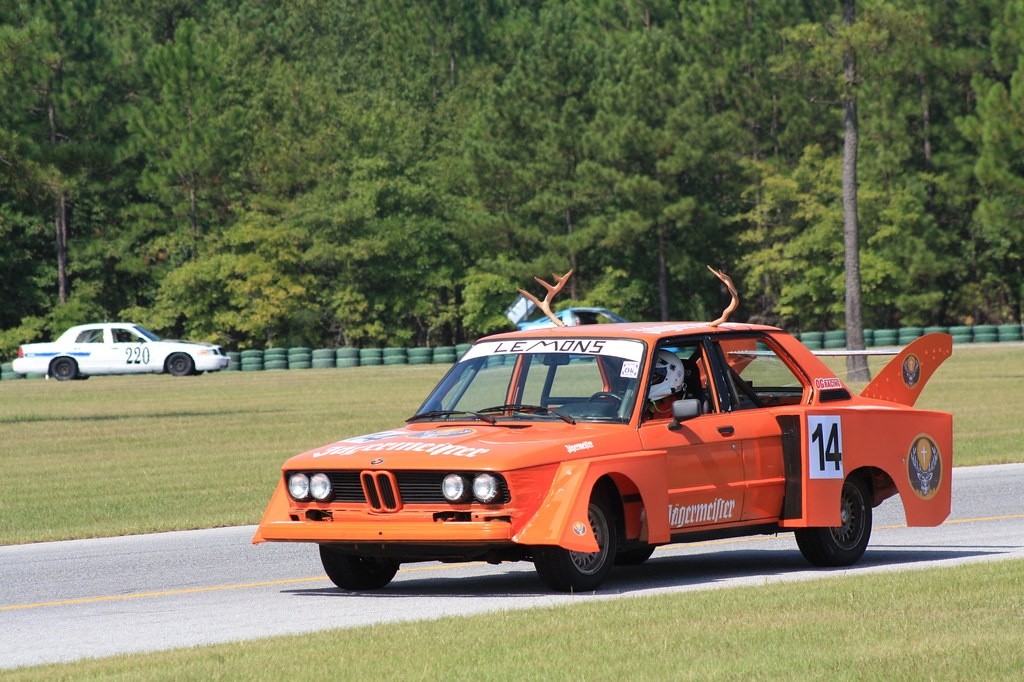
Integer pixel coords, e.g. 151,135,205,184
252,267,953,595
12,323,231,381
518,308,679,356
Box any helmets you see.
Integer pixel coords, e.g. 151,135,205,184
647,350,684,403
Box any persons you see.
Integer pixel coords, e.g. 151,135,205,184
641,349,684,420
